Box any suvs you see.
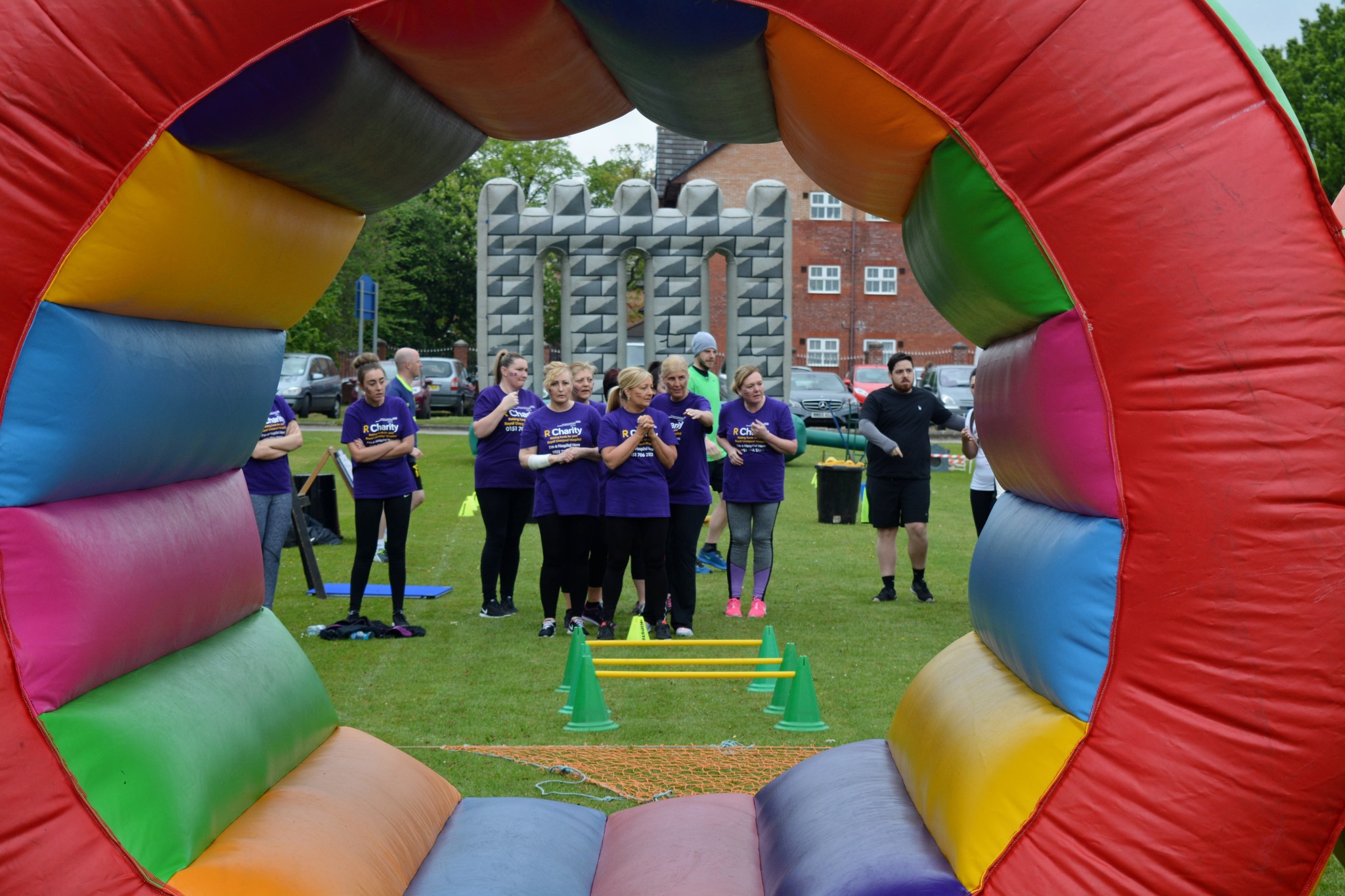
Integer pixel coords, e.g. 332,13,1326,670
921,364,977,430
418,357,478,417
275,353,342,418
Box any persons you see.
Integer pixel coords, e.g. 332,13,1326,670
473,349,547,619
857,352,967,603
686,331,729,575
920,361,934,378
373,347,425,562
243,395,303,612
341,352,412,628
519,354,713,641
716,364,798,618
961,367,998,536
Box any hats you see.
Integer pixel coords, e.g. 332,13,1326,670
692,332,717,356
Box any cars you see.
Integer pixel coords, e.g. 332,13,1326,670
843,365,927,411
719,359,860,429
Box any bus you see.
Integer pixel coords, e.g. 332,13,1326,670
349,360,433,418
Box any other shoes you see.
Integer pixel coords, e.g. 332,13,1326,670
633,602,640,614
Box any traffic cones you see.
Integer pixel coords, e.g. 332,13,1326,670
458,501,474,517
810,472,817,488
563,655,620,732
774,657,829,733
465,491,479,511
557,644,612,715
625,615,651,641
554,627,604,693
762,643,798,714
746,625,782,692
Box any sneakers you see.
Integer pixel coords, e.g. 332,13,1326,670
747,596,766,617
374,549,390,562
872,588,896,602
698,548,726,570
479,599,508,618
726,598,742,617
538,620,556,637
391,611,409,626
566,620,589,636
676,627,692,637
501,596,519,615
654,621,672,640
597,621,615,639
347,610,359,619
582,603,617,627
666,598,672,613
910,581,934,602
695,557,712,574
645,621,652,631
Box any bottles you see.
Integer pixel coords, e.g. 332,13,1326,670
350,630,369,640
306,625,327,636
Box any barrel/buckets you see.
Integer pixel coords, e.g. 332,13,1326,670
814,464,862,526
